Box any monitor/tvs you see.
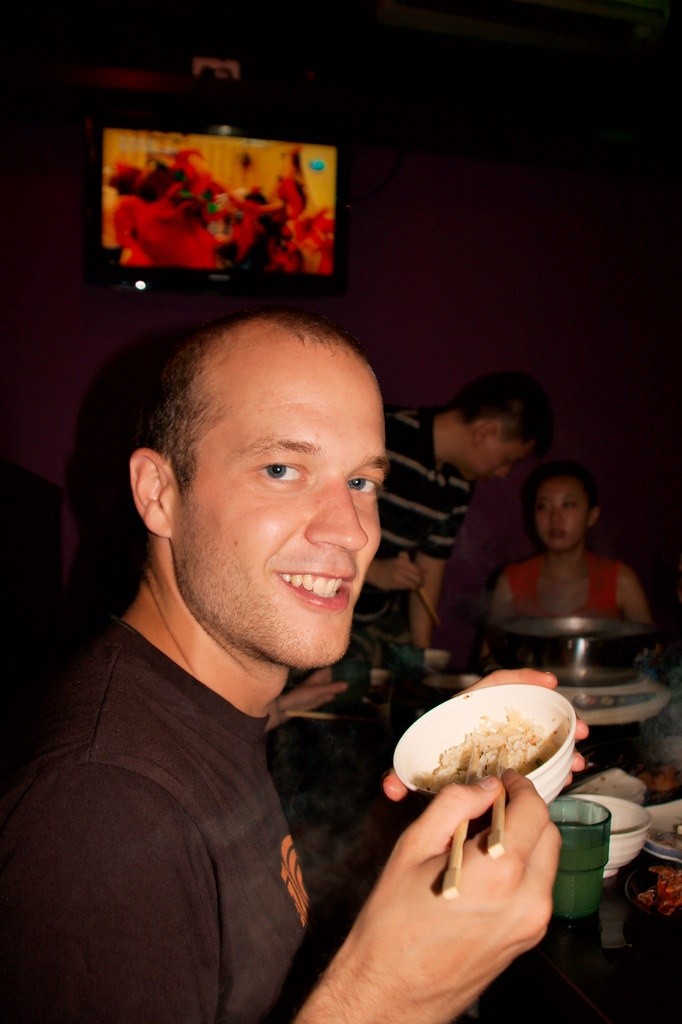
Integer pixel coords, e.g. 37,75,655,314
79,113,351,294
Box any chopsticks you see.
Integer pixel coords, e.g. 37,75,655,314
441,745,505,900
400,552,440,629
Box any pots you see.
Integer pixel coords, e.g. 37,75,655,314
485,615,656,686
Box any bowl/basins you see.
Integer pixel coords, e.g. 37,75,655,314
393,682,577,807
568,794,652,879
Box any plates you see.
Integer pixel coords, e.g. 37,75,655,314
624,863,682,927
643,799,682,862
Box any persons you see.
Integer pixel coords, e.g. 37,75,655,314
264,366,551,732
0,304,563,1024
488,460,653,624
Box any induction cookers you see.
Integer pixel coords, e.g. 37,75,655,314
556,677,673,728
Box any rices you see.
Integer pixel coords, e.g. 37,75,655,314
407,711,559,793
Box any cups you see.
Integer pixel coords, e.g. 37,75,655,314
545,796,612,921
